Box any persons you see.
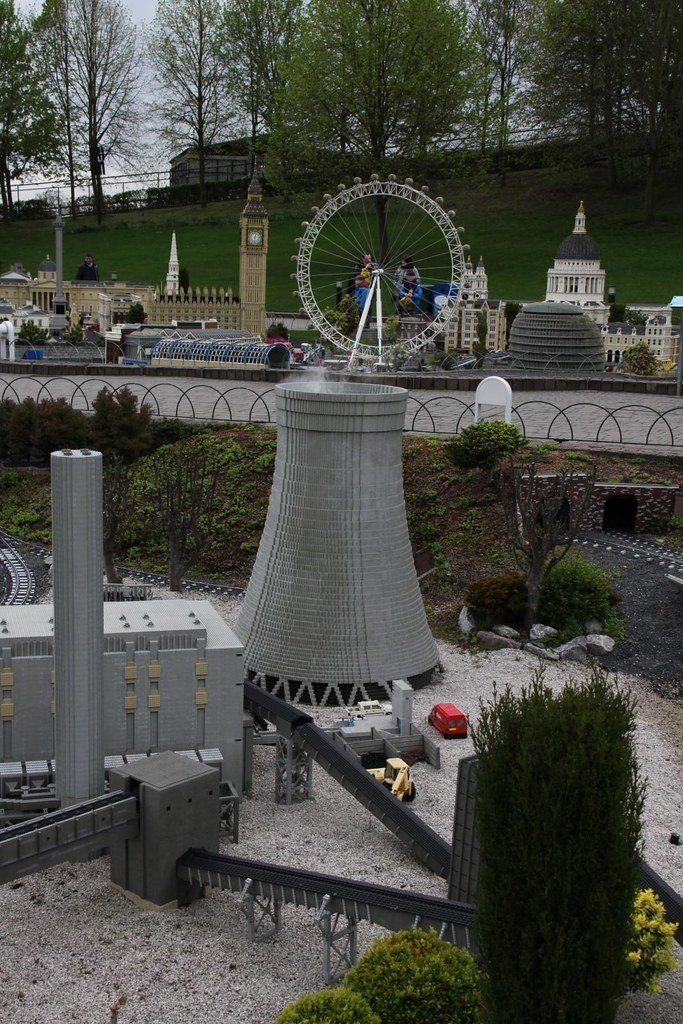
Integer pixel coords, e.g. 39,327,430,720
354,275,373,329
346,251,379,317
398,254,423,319
75,253,99,282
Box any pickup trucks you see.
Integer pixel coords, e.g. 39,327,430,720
344,700,393,717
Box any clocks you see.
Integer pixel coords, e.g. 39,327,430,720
248,231,261,246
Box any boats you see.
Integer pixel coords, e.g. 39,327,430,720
317,359,349,372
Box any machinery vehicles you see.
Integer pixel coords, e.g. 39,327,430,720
366,757,416,802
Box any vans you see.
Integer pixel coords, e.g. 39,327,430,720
21,348,44,360
428,703,467,739
